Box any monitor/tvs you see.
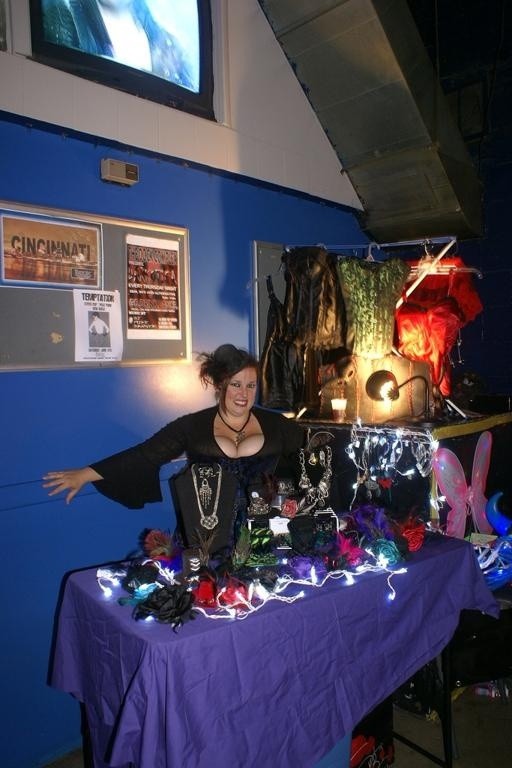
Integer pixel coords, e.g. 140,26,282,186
29,0,221,123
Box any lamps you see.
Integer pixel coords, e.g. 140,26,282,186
367,370,437,428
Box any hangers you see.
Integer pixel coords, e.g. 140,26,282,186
364,240,483,276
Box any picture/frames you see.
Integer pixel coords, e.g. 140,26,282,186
0,200,191,372
255,240,285,361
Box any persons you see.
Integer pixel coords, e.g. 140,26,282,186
89,311,111,346
42,344,310,563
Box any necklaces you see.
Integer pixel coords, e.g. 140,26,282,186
190,461,223,531
217,407,252,446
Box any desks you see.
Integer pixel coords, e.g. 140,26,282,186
291,414,512,520
47,531,502,768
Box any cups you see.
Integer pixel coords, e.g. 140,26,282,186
330,398,348,421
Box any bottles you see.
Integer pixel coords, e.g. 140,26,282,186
458,681,509,704
